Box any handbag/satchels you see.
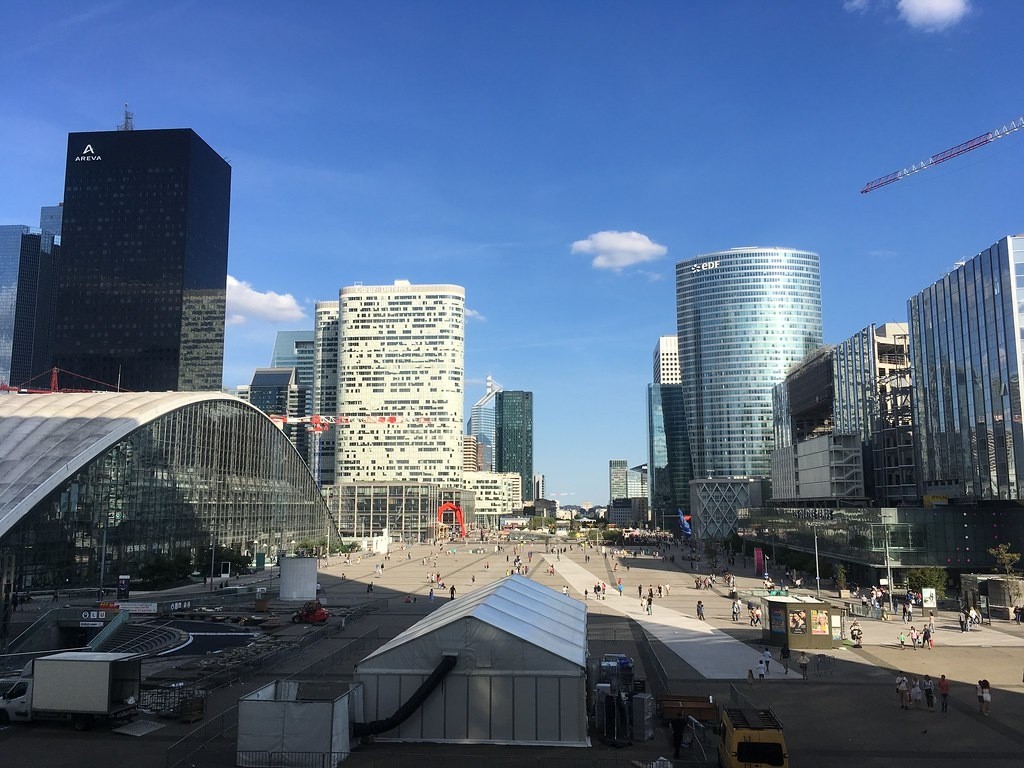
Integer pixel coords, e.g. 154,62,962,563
930,640,934,646
896,688,899,693
983,693,991,703
646,607,648,611
748,678,753,684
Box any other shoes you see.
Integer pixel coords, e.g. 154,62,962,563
648,613,653,615
941,708,947,713
733,618,738,621
803,675,808,680
785,670,788,675
961,629,965,633
928,707,934,712
983,710,990,717
901,704,904,709
901,647,905,650
905,705,909,710
921,646,924,648
914,646,917,650
980,709,982,712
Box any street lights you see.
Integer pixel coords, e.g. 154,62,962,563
875,513,893,611
808,521,823,596
764,554,770,574
660,509,665,537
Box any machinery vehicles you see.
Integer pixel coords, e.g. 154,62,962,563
291,598,330,625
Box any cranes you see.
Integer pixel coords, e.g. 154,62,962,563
1,368,130,393
264,414,431,494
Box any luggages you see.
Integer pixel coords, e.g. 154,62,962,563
928,647,931,649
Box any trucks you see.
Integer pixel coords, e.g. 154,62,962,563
1,653,144,731
713,703,791,767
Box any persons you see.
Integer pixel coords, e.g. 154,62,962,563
355,546,358,553
367,582,374,594
24,590,35,604
317,582,327,594
356,555,361,564
345,554,353,567
368,524,799,578
201,576,207,588
236,572,239,580
472,575,476,587
341,573,347,580
405,596,417,603
426,571,448,590
429,588,434,601
12,591,19,612
562,580,1024,718
218,581,230,588
51,588,59,602
449,585,457,600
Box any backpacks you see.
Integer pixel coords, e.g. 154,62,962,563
783,647,790,659
